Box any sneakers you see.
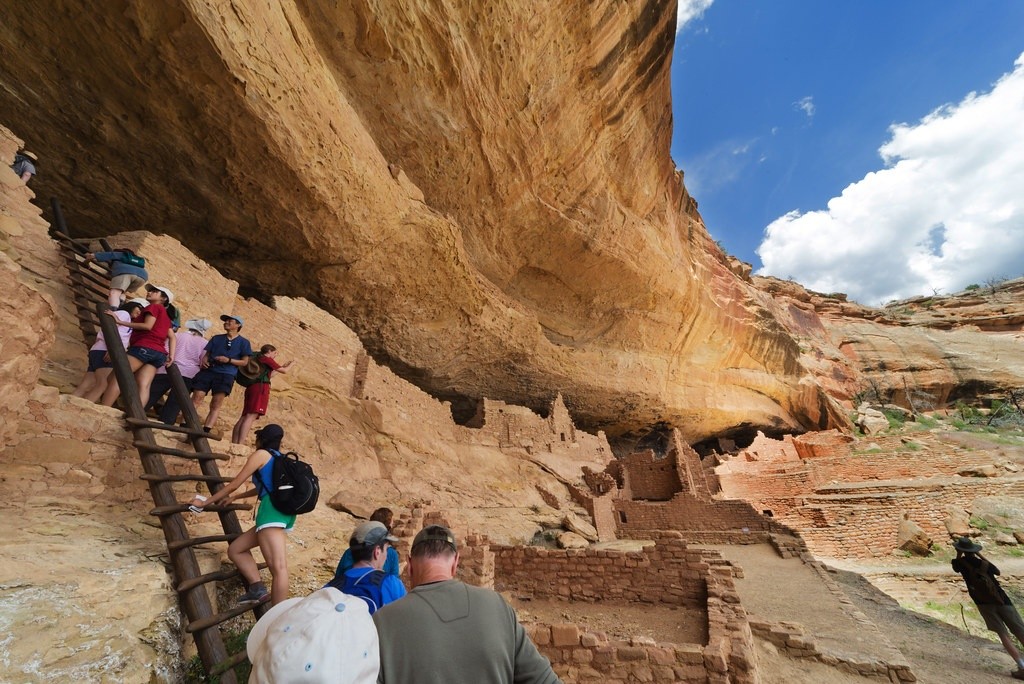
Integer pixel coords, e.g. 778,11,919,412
237,580,271,604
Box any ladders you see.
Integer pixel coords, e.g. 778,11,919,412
50,195,168,418
95,300,283,684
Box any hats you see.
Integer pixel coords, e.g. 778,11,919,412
254,424,284,442
114,248,134,254
411,524,458,551
954,538,982,552
220,314,243,326
349,520,399,549
185,318,211,337
246,587,379,684
23,150,39,161
126,297,150,307
145,283,173,303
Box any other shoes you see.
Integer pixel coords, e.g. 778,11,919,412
148,418,165,424
1011,666,1024,679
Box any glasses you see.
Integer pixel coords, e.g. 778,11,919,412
227,340,232,349
138,304,143,309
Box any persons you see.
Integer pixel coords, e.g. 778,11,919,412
320,521,406,615
371,524,566,684
12,152,36,184
334,508,399,577
951,538,1024,681
247,586,381,684
190,423,298,609
71,249,293,445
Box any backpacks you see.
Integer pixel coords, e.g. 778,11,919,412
236,352,270,386
958,557,1004,606
329,568,388,614
121,250,145,267
252,447,319,515
